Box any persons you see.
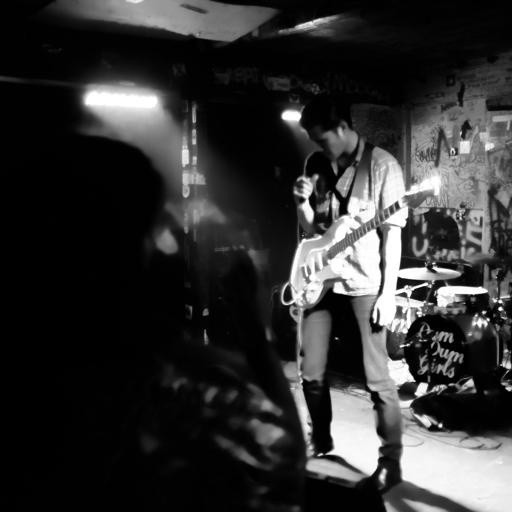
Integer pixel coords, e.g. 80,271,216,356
291,95,409,496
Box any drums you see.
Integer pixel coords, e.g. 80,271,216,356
436,286,490,316
498,296,512,324
404,314,503,392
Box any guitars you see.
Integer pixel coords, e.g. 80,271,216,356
290,189,434,308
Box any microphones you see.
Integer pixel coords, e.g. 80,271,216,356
299,151,324,204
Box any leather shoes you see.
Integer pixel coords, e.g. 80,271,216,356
357,459,404,498
304,440,333,459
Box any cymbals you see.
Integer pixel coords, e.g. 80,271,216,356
396,297,433,308
398,267,462,281
